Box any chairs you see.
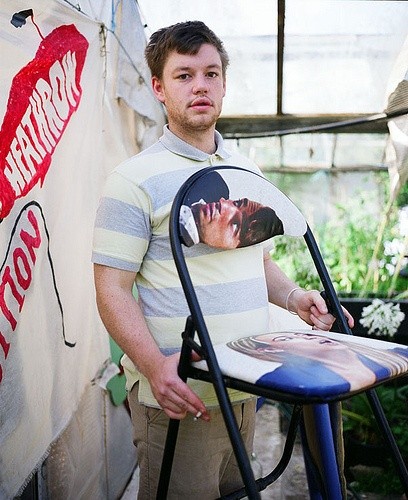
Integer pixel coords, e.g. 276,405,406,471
154,164,408,500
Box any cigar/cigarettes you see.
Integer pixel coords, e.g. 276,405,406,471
193,404,207,421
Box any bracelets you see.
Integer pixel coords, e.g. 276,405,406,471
286,287,307,315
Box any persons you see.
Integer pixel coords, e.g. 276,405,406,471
226,329,408,396
91,20,354,500
179,171,284,250
